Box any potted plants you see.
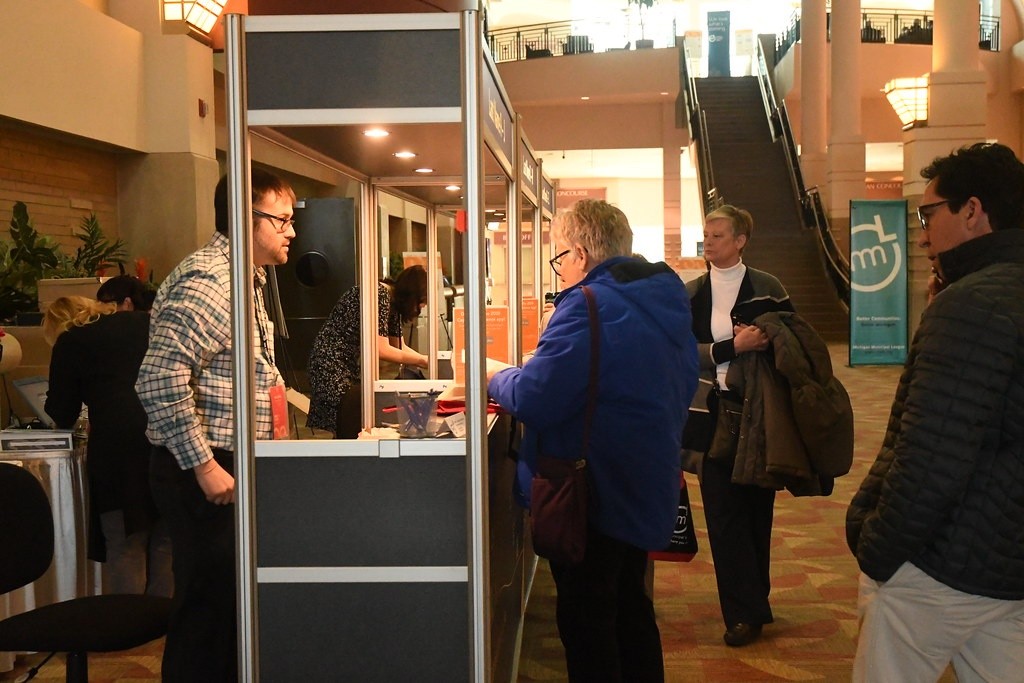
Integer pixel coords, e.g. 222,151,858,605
627,0,654,49
0,201,162,327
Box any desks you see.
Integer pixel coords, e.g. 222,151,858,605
0,409,103,674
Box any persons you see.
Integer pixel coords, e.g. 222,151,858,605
486,199,700,683
42,295,173,597
306,265,428,439
845,141,1024,683
685,204,796,646
97,274,156,313
134,168,297,683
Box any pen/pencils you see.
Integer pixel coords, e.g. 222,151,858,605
395,389,438,436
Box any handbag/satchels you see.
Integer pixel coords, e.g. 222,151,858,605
528,455,591,561
705,393,745,472
647,470,698,562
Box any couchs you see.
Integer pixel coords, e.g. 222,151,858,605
605,41,631,52
526,45,553,58
562,36,594,55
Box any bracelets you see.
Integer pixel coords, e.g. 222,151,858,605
197,467,216,476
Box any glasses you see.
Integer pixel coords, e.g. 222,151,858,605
548,248,588,275
730,312,753,329
251,208,296,232
915,199,963,231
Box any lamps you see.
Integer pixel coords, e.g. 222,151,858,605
163,0,228,34
884,76,929,124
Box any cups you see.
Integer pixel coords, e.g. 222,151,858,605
394,394,438,437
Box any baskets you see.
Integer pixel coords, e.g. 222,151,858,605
392,391,440,439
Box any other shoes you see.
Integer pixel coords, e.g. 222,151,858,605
723,615,762,646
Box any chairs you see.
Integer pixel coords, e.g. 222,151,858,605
0,462,176,683
